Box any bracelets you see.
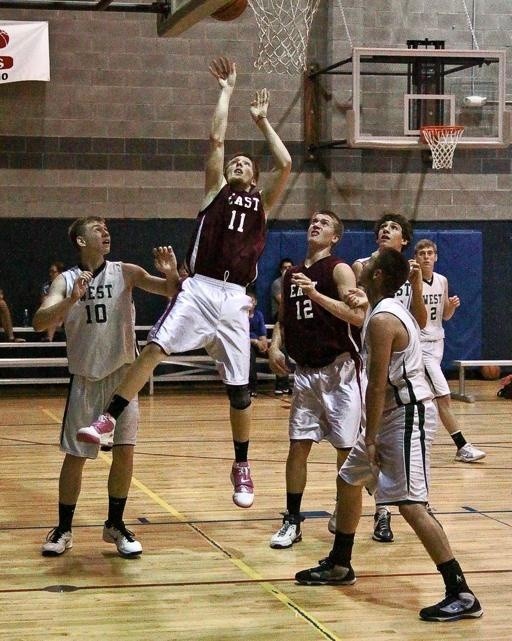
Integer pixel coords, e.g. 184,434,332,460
365,442,375,447
256,114,266,124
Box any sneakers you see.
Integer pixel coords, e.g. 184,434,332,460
229,461,255,508
77,414,116,447
274,382,290,394
373,510,394,542
420,588,483,622
454,443,486,463
295,557,356,584
40,338,50,355
271,514,302,548
328,509,337,533
41,527,72,557
103,520,142,556
250,386,257,396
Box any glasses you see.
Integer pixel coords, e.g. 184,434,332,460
48,269,59,273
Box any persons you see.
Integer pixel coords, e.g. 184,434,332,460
0,290,27,343
294,247,484,623
40,262,64,342
271,257,295,321
244,292,291,396
269,209,365,551
344,214,442,542
35,215,179,557
412,239,486,462
78,58,292,507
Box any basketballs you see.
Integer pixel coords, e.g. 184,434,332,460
481,365,501,380
210,0,247,22
